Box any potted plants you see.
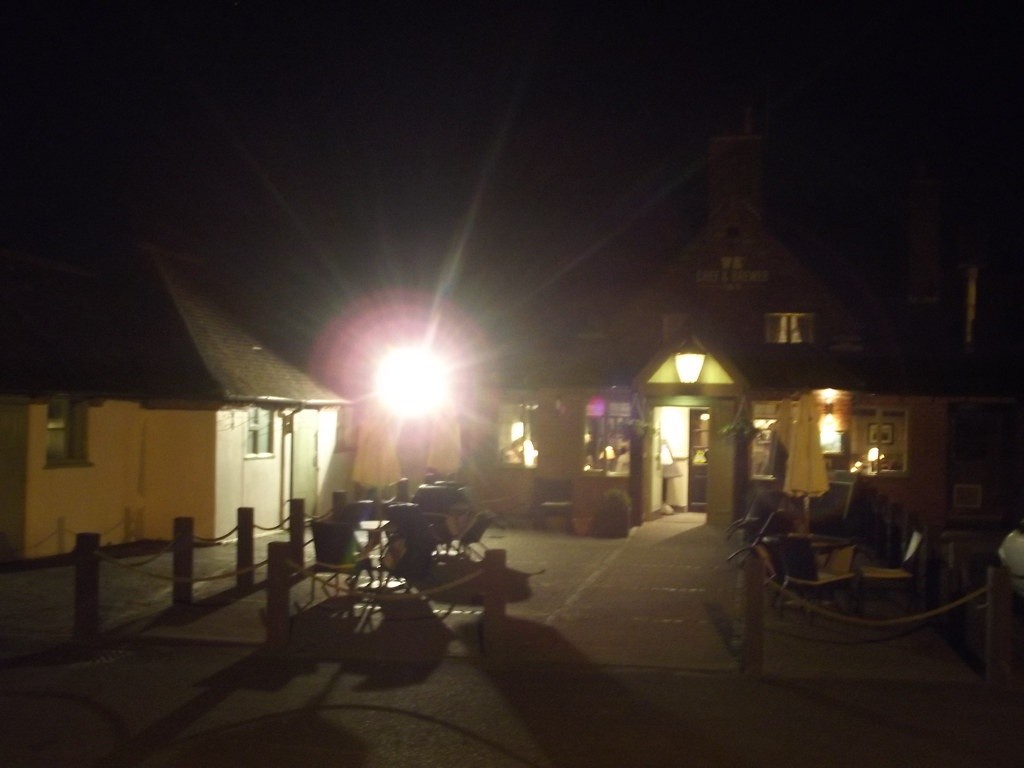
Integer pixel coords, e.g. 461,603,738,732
609,393,655,447
717,394,762,450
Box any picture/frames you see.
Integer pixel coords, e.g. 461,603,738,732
867,421,895,444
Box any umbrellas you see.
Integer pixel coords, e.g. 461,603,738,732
423,404,462,480
768,384,830,530
349,398,403,522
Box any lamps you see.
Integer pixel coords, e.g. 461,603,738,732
675,335,707,384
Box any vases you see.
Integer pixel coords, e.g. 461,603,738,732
540,502,572,531
570,517,596,534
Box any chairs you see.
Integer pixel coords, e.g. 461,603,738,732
724,477,1023,623
306,479,496,617
